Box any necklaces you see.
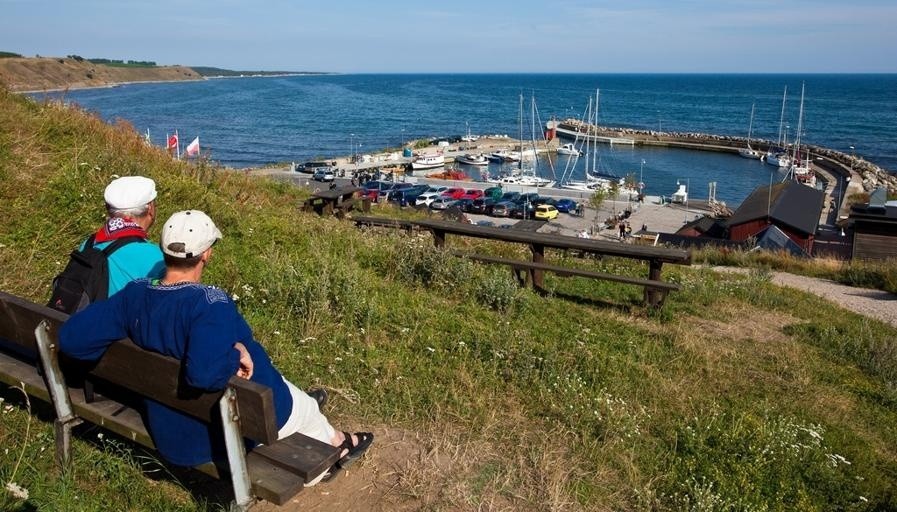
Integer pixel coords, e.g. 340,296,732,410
739,82,817,184
560,89,639,196
488,86,558,187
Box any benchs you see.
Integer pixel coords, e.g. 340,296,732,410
169,133,177,150
186,137,199,156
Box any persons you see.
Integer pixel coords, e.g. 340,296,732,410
80,175,328,411
575,202,580,215
328,168,393,191
619,210,647,236
581,201,585,216
57,210,374,489
578,230,589,239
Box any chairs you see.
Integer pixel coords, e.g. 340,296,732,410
307,389,327,409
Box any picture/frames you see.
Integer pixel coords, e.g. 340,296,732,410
310,184,362,215
352,216,692,306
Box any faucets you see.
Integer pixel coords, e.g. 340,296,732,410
321,431,373,482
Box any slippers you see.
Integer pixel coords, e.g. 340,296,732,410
47,232,147,314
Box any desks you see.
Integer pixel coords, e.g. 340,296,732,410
837,175,850,221
676,179,689,223
850,146,855,176
840,228,845,259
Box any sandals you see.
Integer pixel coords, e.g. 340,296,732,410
304,161,332,172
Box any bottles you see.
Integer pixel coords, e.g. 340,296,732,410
104,176,157,210
161,210,223,259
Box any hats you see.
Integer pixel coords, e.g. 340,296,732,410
358,180,576,220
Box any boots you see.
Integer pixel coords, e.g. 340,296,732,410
312,171,334,182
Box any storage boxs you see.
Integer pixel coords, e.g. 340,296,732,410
446,252,679,309
337,200,354,214
303,197,320,211
0,292,342,512
360,196,371,212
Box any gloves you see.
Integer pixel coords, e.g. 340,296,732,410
426,168,473,182
455,153,489,165
412,151,444,170
382,167,404,172
